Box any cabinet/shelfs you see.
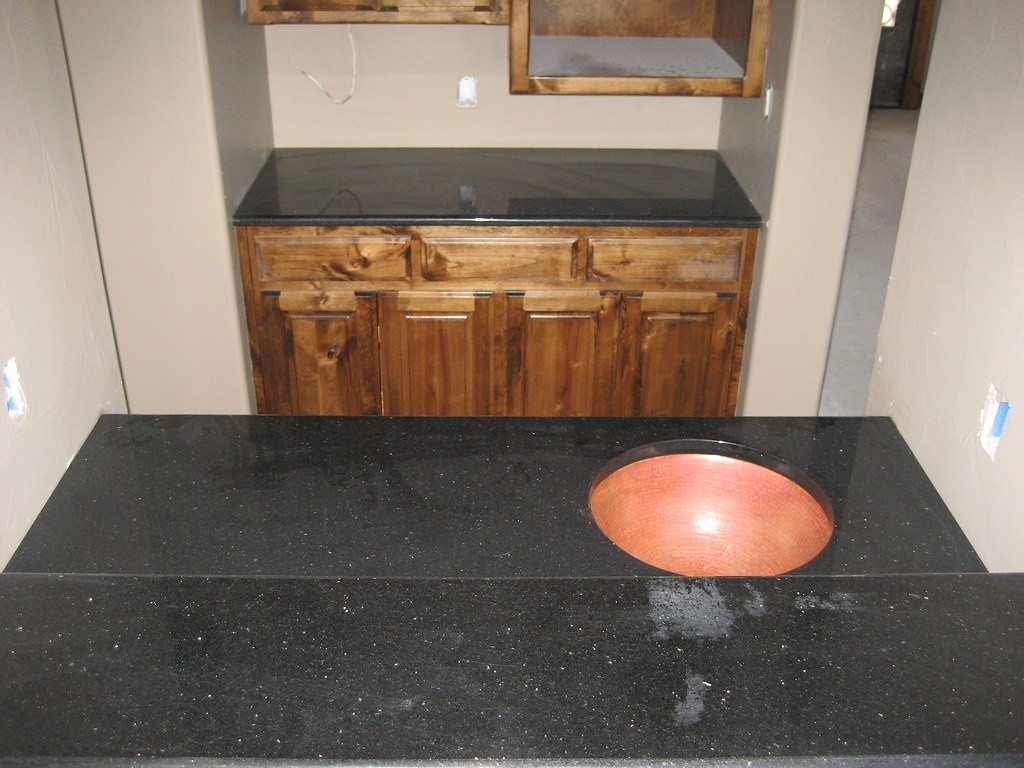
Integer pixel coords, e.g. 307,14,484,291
247,0,773,98
233,220,760,416
